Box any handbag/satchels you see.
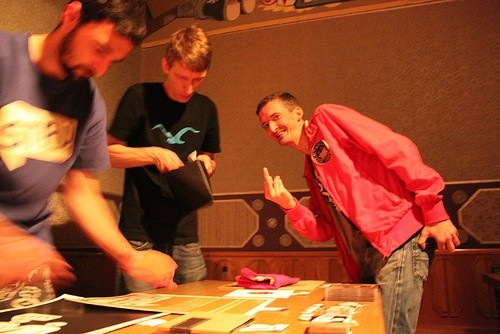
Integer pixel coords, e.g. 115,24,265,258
159,161,212,214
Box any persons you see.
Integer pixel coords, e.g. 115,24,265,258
256,92,461,334
106,25,222,297
0,0,178,309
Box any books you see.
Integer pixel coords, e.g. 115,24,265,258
324,284,378,301
157,312,254,334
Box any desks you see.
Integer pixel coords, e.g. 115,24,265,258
0,280,387,334
